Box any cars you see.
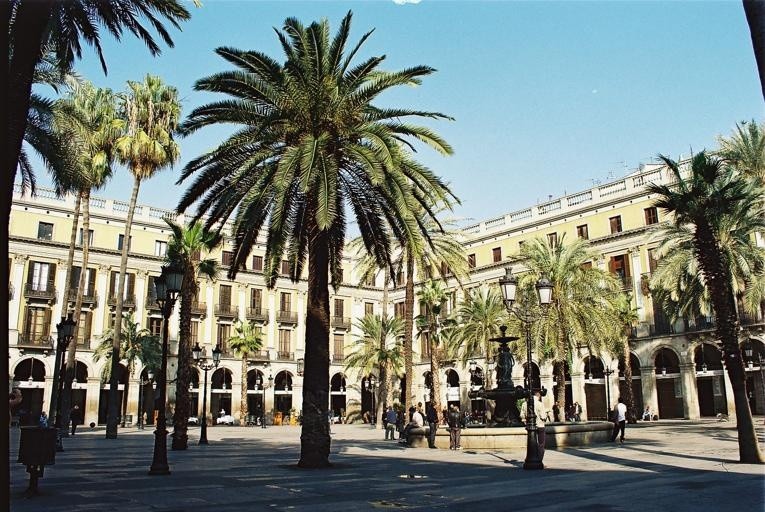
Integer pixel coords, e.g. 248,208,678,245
160,409,357,429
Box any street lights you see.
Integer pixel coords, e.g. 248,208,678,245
145,367,156,427
145,249,186,480
364,375,381,429
496,264,556,470
191,341,223,448
467,356,494,425
602,365,614,421
51,311,79,430
255,374,276,430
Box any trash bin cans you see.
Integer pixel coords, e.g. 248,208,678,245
273,412,282,425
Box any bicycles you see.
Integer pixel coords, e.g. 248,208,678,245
540,410,552,423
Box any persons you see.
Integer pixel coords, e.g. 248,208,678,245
496,347,505,388
70,404,80,434
9,388,23,428
219,408,226,416
504,346,515,383
255,403,263,426
520,390,547,461
609,397,628,441
546,401,582,421
382,401,480,450
641,405,654,421
